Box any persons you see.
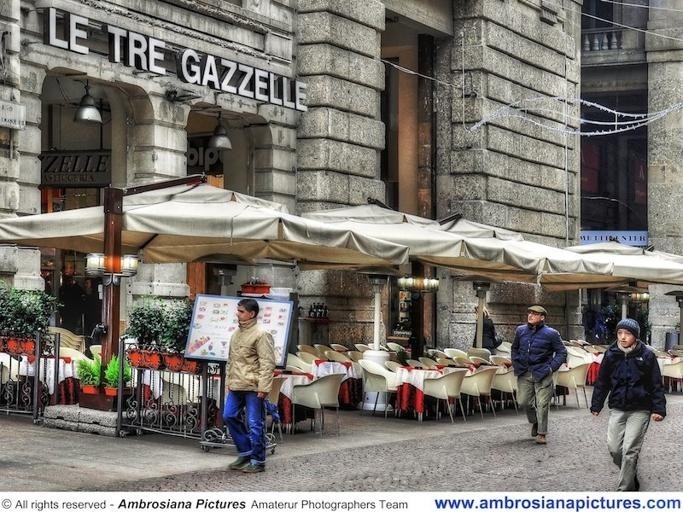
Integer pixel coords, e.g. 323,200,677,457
58,275,87,331
222,298,276,473
472,305,503,355
590,317,667,492
84,277,93,296
511,305,567,444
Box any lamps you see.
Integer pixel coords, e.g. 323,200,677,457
74,77,102,126
209,111,234,154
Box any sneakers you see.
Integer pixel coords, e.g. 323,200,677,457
535,434,546,444
242,464,265,474
228,456,250,470
531,423,538,437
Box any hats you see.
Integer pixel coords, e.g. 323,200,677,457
527,304,548,315
615,318,641,339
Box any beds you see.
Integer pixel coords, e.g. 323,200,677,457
587,351,609,387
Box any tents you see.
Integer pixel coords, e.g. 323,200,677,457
298,198,614,282
0,174,410,410
563,240,683,316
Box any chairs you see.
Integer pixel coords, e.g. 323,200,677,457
423,368,465,423
553,365,589,410
323,349,346,362
345,352,364,364
443,347,468,362
499,366,521,417
465,346,490,361
330,341,351,353
312,344,331,359
437,358,458,371
462,365,498,420
289,373,344,441
492,354,510,365
497,342,520,357
357,360,392,418
297,342,314,362
355,343,372,354
563,346,584,362
428,347,448,361
388,358,403,375
470,355,491,371
405,360,429,374
455,356,474,371
418,357,435,370
294,350,321,373
384,342,407,353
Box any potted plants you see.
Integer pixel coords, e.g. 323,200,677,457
74,354,103,396
104,354,137,395
